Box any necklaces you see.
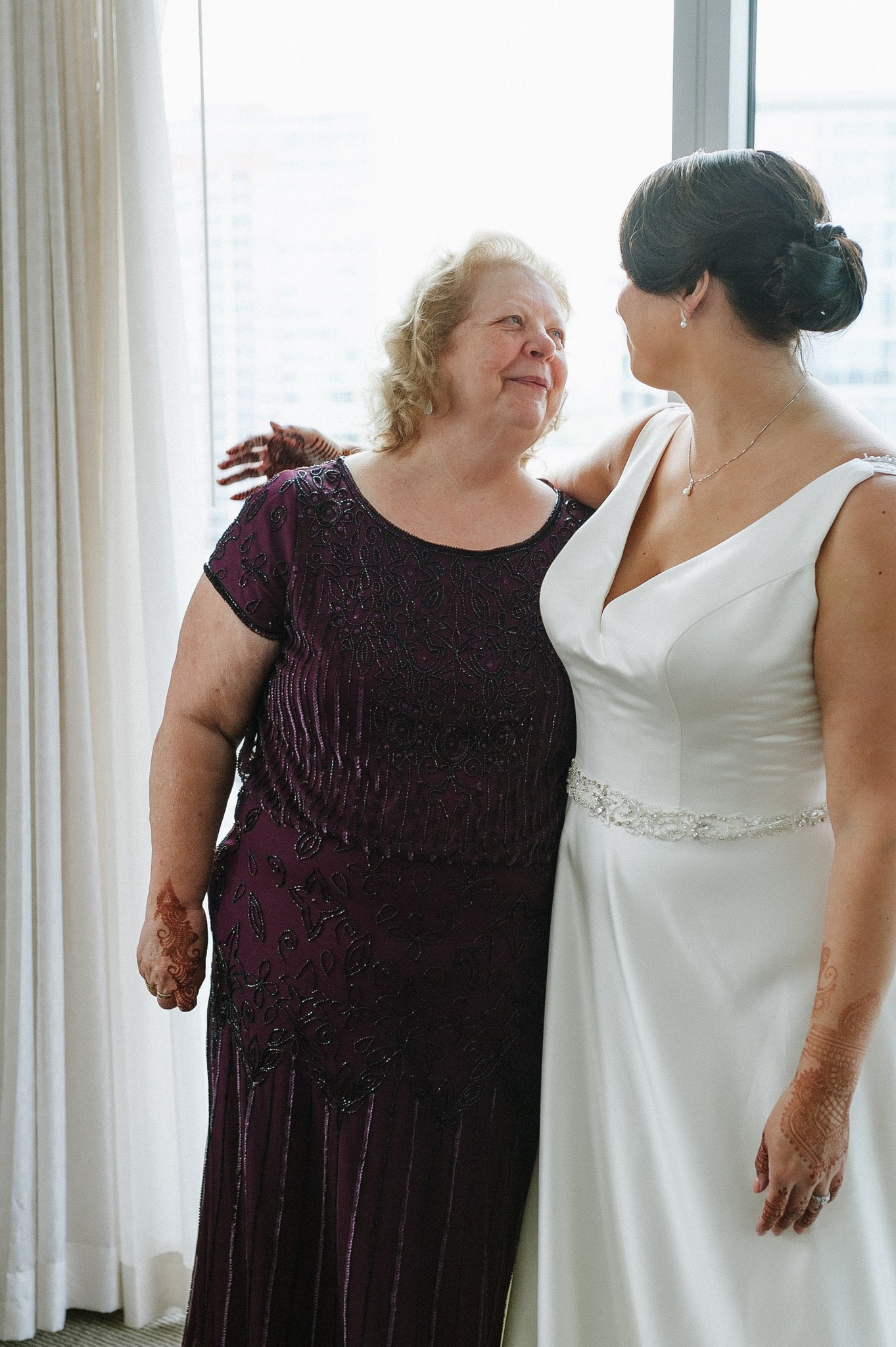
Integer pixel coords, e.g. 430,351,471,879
682,371,813,496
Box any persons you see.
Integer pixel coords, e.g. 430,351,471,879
134,224,610,1346
214,143,896,1347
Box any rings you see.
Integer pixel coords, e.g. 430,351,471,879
156,989,174,999
145,980,156,991
812,1189,831,1206
